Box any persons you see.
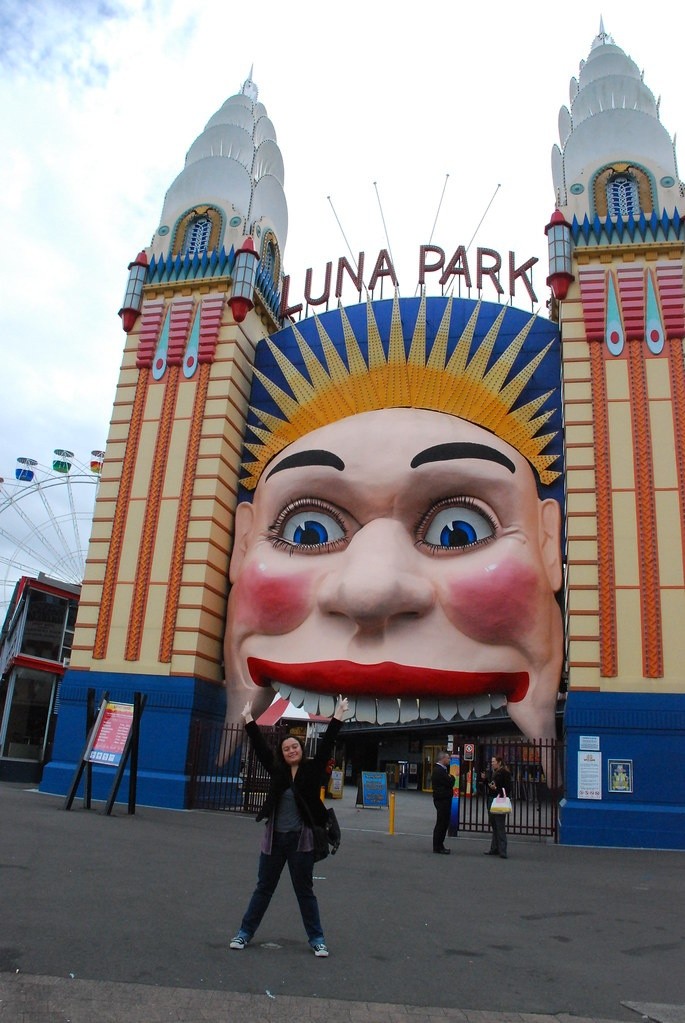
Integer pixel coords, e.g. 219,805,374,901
480,753,513,859
227,694,349,957
431,751,455,855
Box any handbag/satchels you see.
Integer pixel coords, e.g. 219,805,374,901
313,826,330,862
490,787,512,814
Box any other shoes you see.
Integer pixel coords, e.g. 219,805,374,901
230,937,247,949
433,848,450,854
313,943,328,956
500,855,507,858
484,851,499,855
